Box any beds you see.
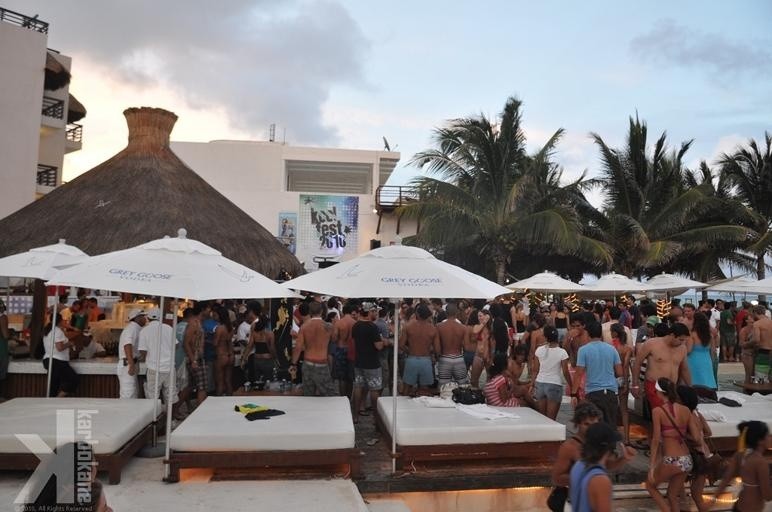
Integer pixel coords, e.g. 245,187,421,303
375,394,568,481
622,389,772,452
167,396,356,484
0,396,162,484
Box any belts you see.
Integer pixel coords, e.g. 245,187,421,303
304,359,330,368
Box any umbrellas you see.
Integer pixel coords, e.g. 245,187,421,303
503,270,772,307
43,229,305,478
279,236,515,473
0,237,94,396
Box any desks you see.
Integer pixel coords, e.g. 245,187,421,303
7,354,163,401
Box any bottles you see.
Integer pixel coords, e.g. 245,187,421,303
240,366,298,393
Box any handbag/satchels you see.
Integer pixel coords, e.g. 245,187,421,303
450,385,486,405
685,440,707,482
545,484,573,512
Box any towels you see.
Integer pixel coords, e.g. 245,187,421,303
457,401,522,421
698,406,727,425
415,395,457,410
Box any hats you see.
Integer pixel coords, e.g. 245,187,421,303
583,422,617,465
146,309,160,321
539,300,551,308
127,308,148,321
